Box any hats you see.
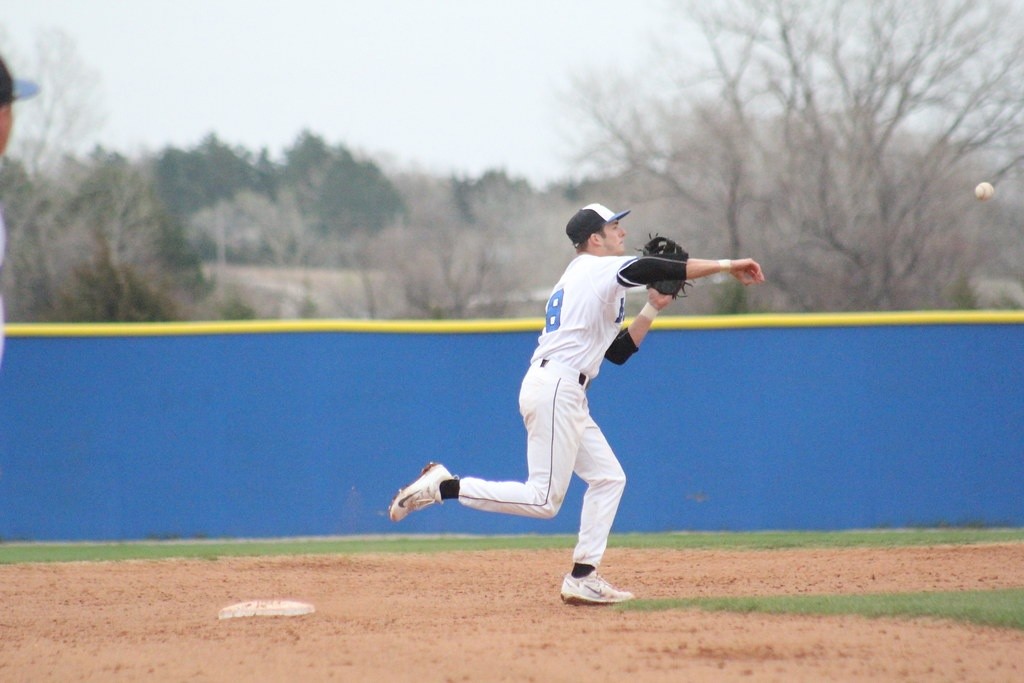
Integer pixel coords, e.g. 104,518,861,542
0,61,40,107
565,203,631,246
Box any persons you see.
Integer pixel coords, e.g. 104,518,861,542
0,59,39,157
388,201,766,606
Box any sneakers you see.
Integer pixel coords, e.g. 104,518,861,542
389,463,455,522
561,570,633,604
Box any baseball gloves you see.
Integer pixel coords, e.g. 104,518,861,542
642,239,688,298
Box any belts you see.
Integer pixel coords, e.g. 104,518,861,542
541,359,591,389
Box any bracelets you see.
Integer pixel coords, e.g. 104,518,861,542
640,302,658,322
718,258,732,275
